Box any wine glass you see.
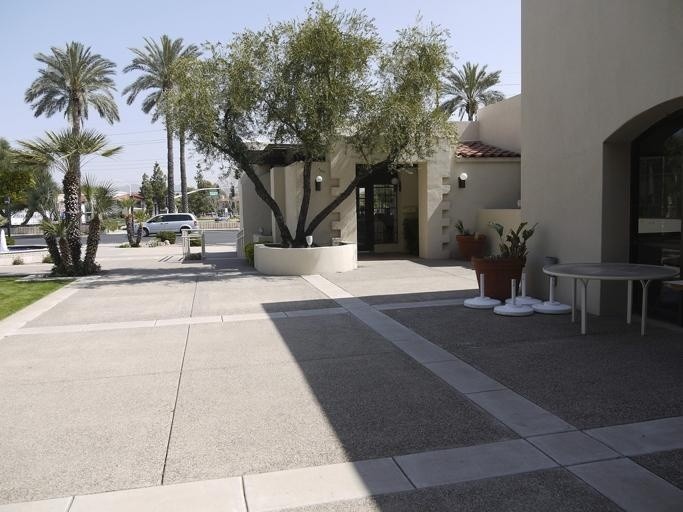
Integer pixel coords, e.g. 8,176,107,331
306,236,313,248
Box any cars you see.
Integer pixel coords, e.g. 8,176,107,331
135,213,199,237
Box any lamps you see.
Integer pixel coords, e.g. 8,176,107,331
315,175,323,191
458,173,468,189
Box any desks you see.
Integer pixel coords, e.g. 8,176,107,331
542,262,681,338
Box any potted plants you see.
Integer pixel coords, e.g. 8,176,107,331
471,219,540,302
454,219,487,260
